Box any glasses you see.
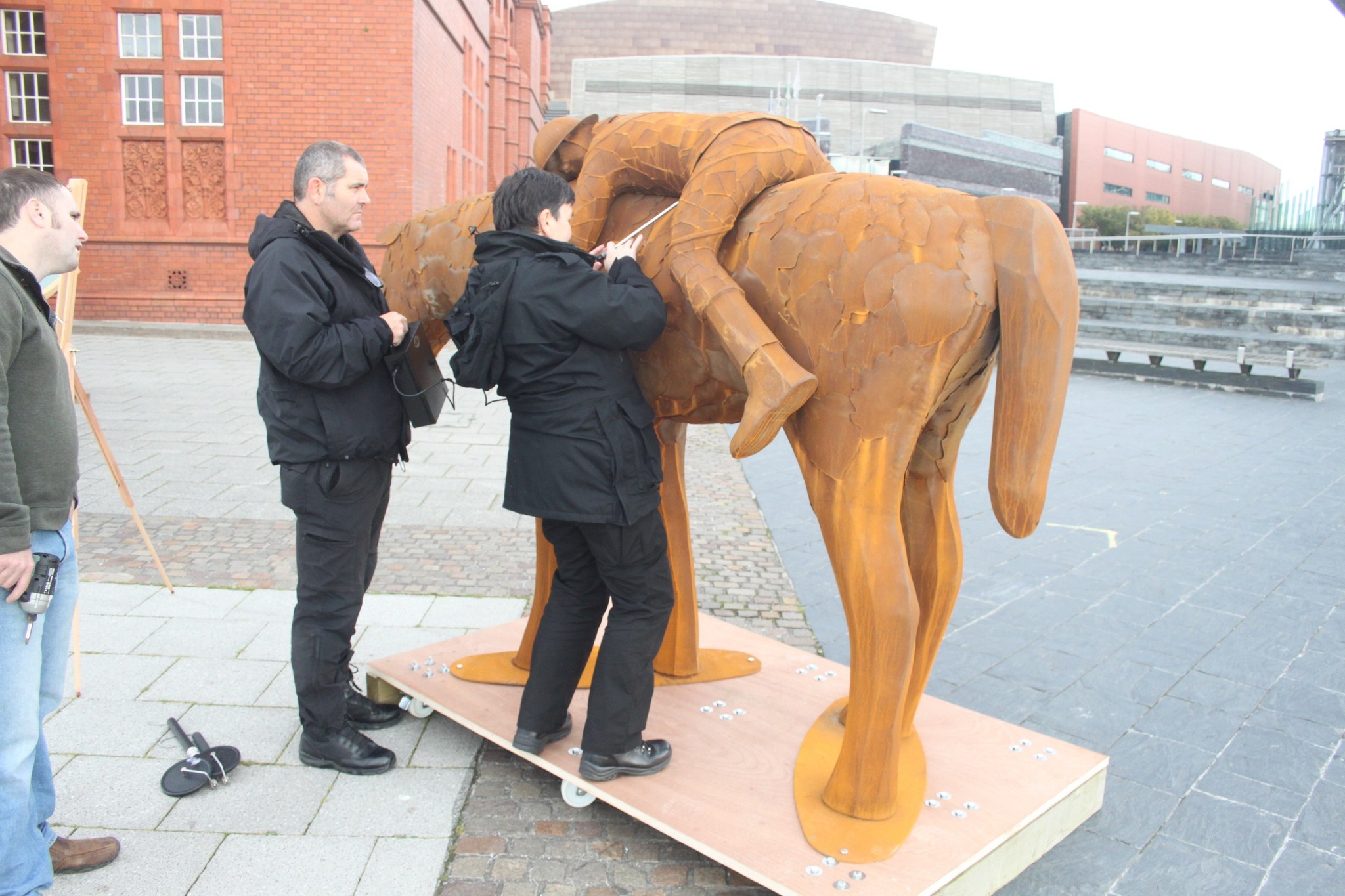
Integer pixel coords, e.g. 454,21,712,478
362,265,384,287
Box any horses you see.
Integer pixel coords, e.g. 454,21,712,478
377,171,1083,819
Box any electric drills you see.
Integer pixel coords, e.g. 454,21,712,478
20,552,60,645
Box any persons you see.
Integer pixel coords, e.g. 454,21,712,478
533,111,836,459
442,169,674,781
0,168,120,896
242,142,411,776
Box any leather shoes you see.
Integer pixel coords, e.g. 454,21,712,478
49,836,121,875
299,721,397,776
346,681,402,729
513,709,573,753
578,739,672,781
